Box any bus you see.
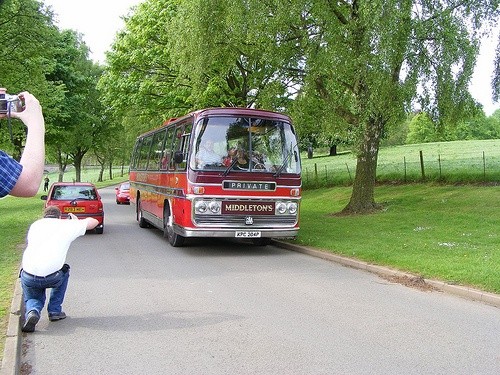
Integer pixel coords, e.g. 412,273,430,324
129,107,304,247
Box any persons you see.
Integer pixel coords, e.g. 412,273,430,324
18,205,99,332
0,81,46,202
44,175,50,192
158,122,270,171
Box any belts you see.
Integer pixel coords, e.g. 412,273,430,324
22,269,62,279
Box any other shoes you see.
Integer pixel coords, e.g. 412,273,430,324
21,310,40,333
49,311,66,321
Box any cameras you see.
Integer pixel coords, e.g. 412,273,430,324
0,93,22,114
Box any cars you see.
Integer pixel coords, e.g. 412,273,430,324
41,182,105,234
115,182,131,205
